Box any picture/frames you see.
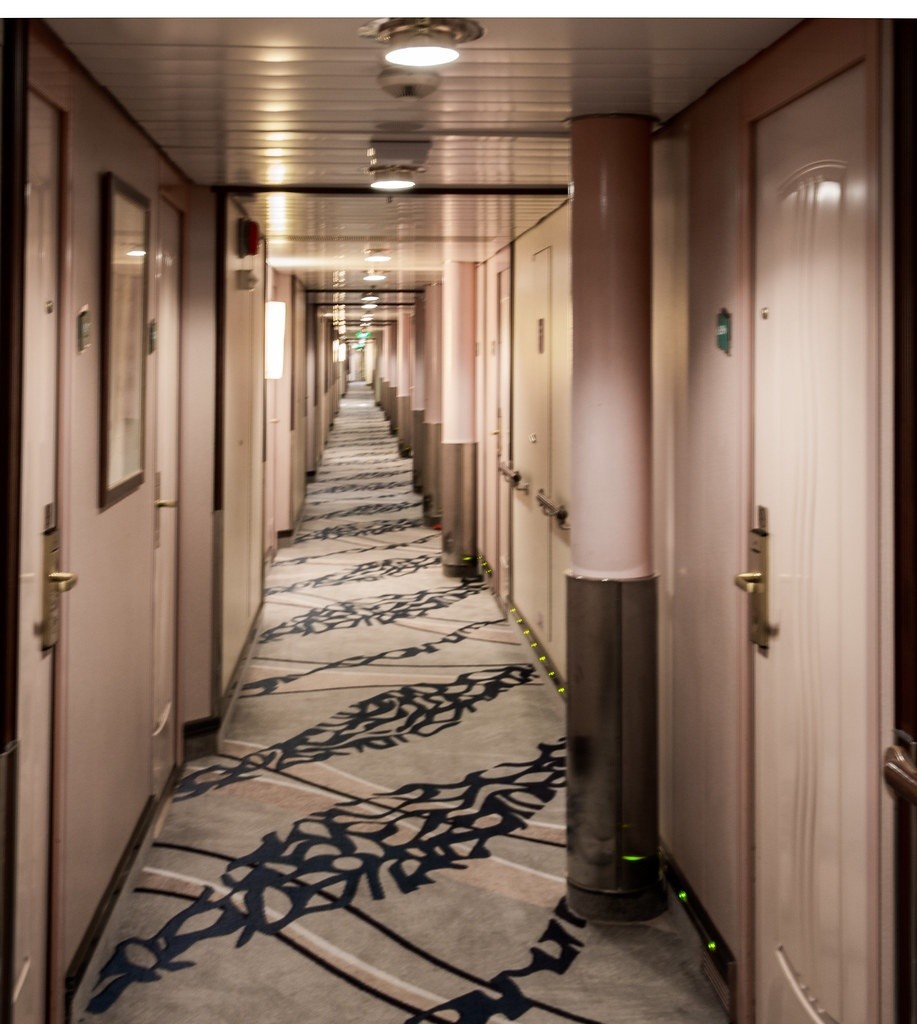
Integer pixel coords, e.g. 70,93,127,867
100,170,152,508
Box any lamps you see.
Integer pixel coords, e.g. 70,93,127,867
360,17,485,192
